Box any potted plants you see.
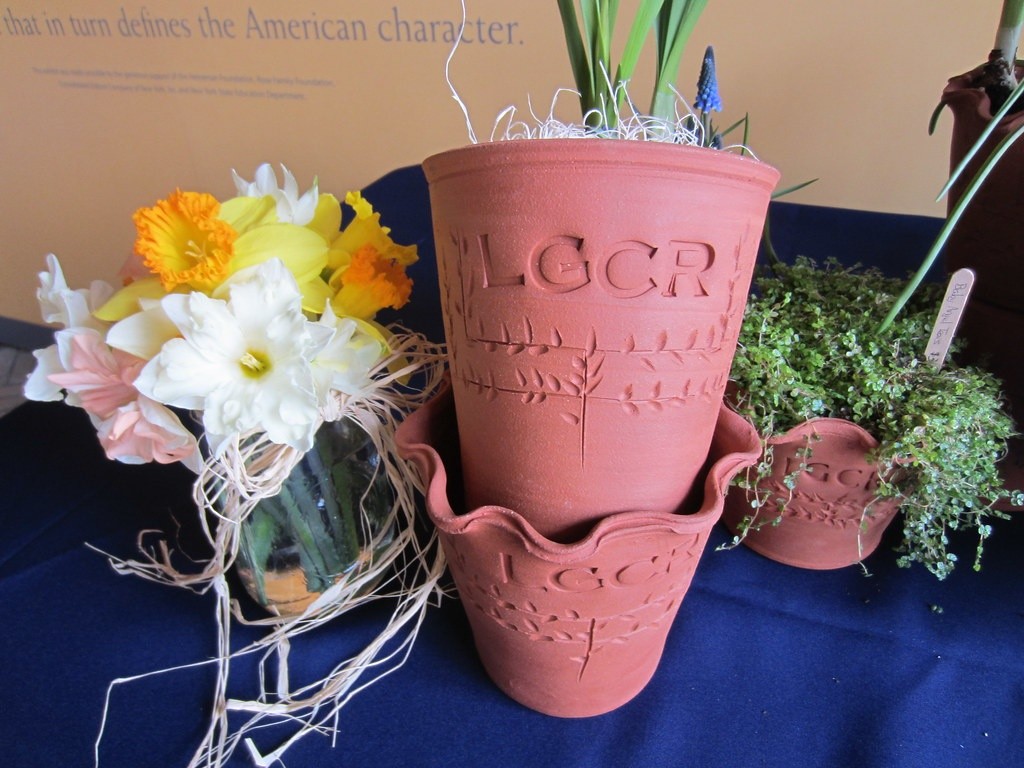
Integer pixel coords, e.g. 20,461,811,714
722,249,1024,585
410,0,781,547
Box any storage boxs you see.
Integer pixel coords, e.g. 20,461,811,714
332,169,958,373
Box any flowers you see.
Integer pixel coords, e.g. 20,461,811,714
19,159,418,476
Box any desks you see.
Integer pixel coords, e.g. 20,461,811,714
0,310,1024,768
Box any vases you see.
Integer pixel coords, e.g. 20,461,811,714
939,58,1024,326
211,415,394,624
387,389,764,725
